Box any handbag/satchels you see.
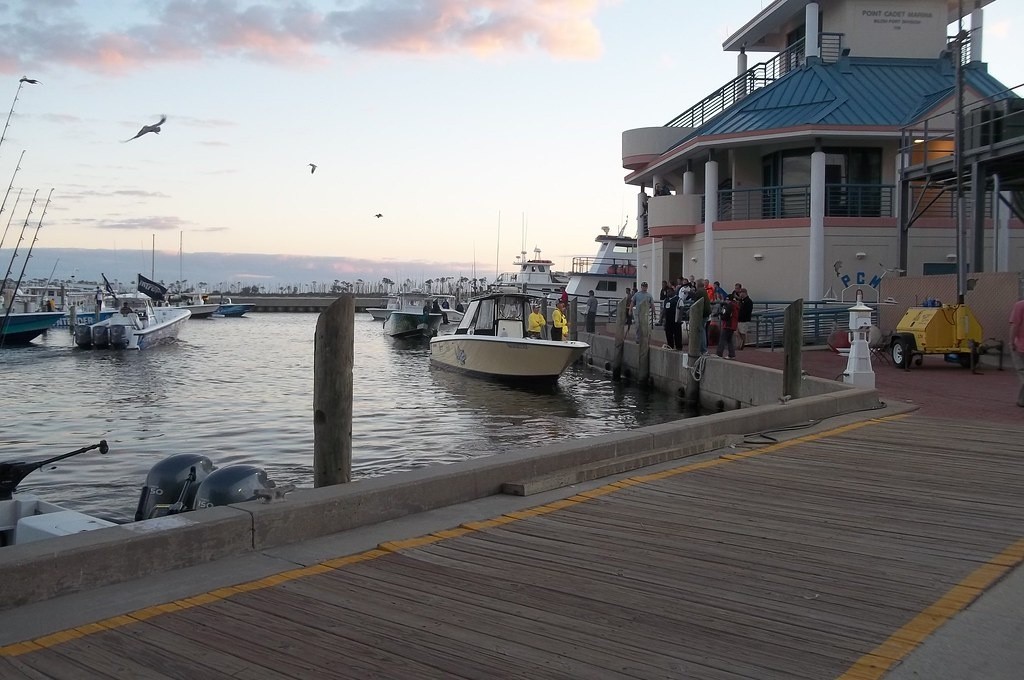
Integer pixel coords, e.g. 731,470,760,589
679,285,691,301
721,305,732,321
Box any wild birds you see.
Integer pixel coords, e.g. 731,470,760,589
375,214,383,218
309,163,317,174
122,114,166,143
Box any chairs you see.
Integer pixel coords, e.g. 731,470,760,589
867,331,893,366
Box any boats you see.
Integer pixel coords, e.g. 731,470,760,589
0,289,67,350
0,487,120,549
218,293,257,318
429,290,591,384
74,298,192,351
530,225,637,319
365,288,464,322
99,271,219,319
382,311,443,341
459,259,571,315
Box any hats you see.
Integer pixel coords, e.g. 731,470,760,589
533,304,540,308
560,285,565,289
632,288,638,292
724,294,733,301
588,290,594,293
740,288,747,293
625,288,631,291
554,298,563,304
641,282,648,285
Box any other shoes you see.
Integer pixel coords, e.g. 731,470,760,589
655,323,662,326
725,356,735,360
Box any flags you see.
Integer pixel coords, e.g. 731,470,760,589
138,274,167,300
103,274,119,301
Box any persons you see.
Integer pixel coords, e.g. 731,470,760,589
584,290,598,334
423,302,430,323
433,298,450,324
550,303,567,341
442,299,450,309
456,301,464,312
46,299,57,312
556,287,568,308
528,305,546,339
120,302,133,314
1008,300,1024,408
625,275,753,361
95,287,103,311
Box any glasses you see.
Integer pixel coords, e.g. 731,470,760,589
643,286,647,287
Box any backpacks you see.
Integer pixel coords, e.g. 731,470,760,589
561,314,568,334
